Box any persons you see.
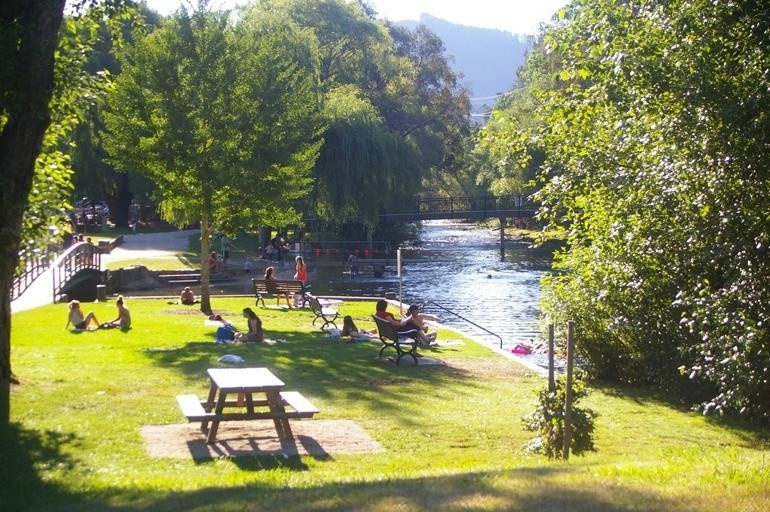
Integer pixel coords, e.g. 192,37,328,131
291,255,308,309
375,299,437,343
255,233,295,265
221,233,232,263
405,303,438,346
88,237,94,258
181,287,198,304
209,250,223,274
341,315,377,337
109,295,131,330
243,256,256,278
230,306,264,344
78,233,86,264
347,250,360,280
65,300,102,330
264,267,291,295
71,235,80,260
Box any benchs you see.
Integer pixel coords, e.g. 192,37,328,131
252,278,311,308
308,295,338,329
176,390,320,444
371,315,418,366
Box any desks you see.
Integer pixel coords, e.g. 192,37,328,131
201,367,293,444
